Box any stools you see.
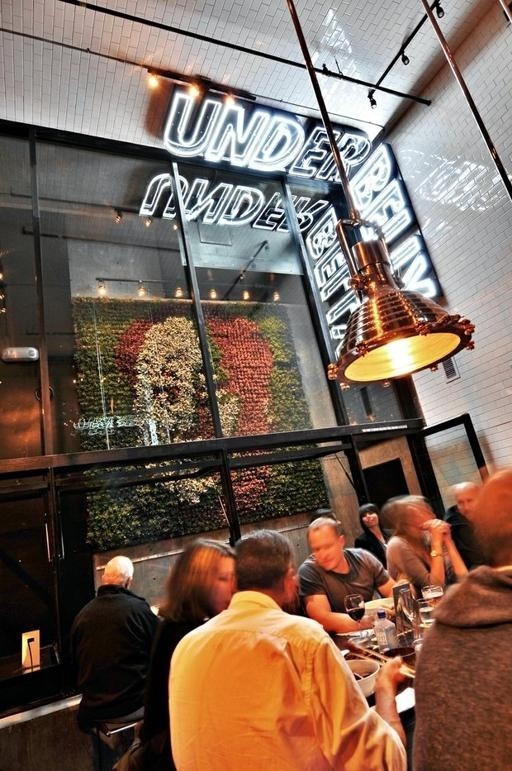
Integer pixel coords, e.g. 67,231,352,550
90,720,147,771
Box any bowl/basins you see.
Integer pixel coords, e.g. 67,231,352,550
343,659,379,698
379,644,418,663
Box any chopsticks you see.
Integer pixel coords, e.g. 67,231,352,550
348,641,420,685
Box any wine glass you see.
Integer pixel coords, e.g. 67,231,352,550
344,593,365,637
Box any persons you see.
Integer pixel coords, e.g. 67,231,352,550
409,465,511,771
295,517,405,636
165,527,412,771
140,538,240,771
309,507,342,528
378,494,469,602
71,554,165,771
353,503,396,572
442,482,494,575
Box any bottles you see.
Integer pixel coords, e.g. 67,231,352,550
373,610,399,651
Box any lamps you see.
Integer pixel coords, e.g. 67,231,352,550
367,0,444,108
95,277,279,302
147,68,256,107
10,191,179,231
221,240,270,298
286,1,476,392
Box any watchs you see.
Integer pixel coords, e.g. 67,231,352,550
430,551,444,559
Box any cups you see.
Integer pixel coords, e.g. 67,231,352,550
419,607,434,624
421,586,445,607
